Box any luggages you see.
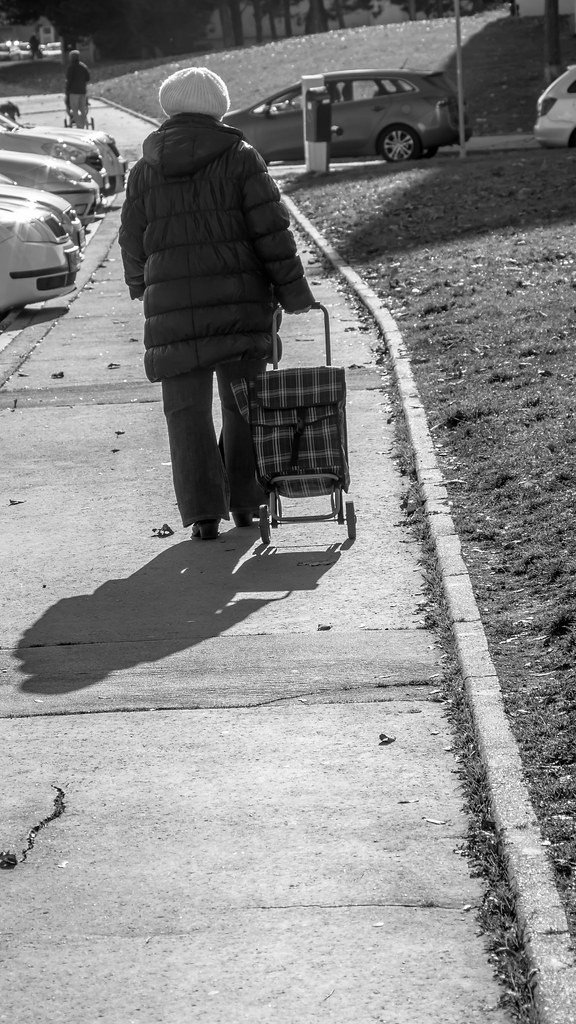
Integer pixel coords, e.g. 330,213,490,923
230,305,359,541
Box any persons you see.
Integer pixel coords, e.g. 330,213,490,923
29,32,40,59
119,64,315,539
65,50,91,130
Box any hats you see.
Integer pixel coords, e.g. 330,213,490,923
158,66,233,121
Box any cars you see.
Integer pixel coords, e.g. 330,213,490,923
1,151,102,229
1,174,89,256
2,200,79,310
219,58,473,168
534,64,576,149
0,115,109,193
0,41,62,61
5,117,129,197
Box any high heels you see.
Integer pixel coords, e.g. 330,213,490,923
190,516,227,540
232,499,264,525
